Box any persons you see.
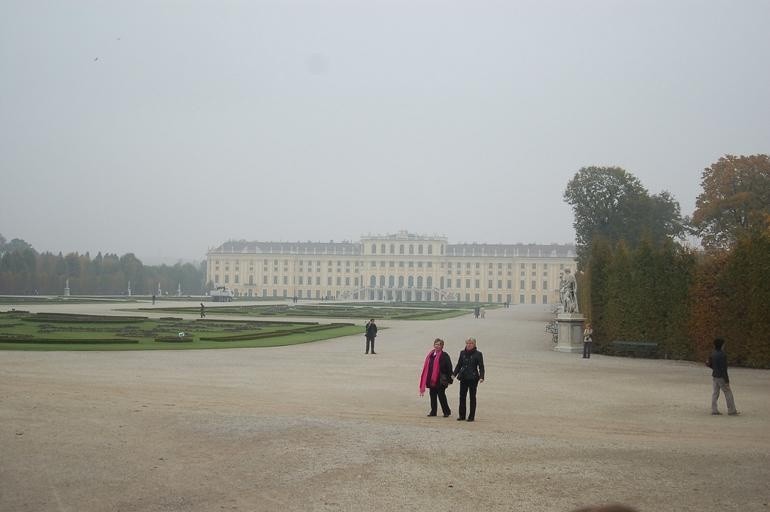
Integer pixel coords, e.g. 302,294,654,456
706,338,742,415
201,303,206,319
365,319,377,354
418,337,454,418
558,266,578,313
583,324,594,359
454,336,486,421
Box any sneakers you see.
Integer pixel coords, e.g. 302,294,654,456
712,410,742,416
427,411,476,423
